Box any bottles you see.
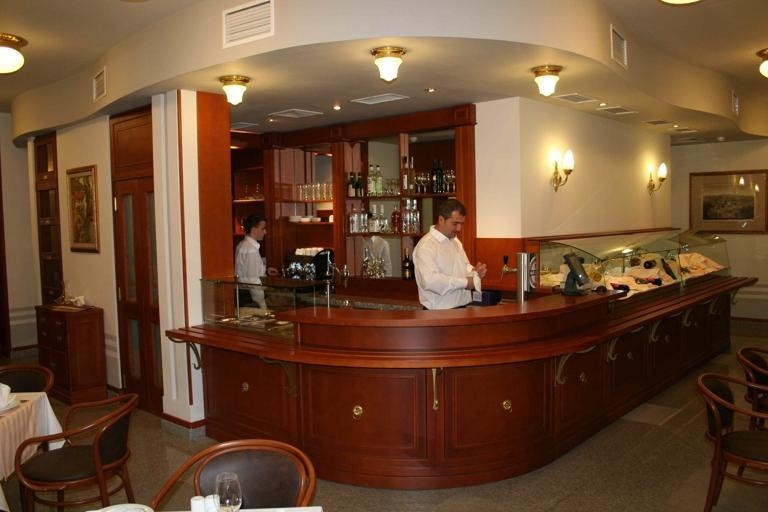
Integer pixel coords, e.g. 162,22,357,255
355,171,363,196
404,199,414,232
347,171,356,197
411,200,421,233
402,155,411,195
391,206,400,233
251,183,264,199
346,202,368,233
432,159,443,193
366,204,389,233
409,156,415,193
661,258,677,280
367,164,377,196
295,182,332,200
402,247,415,280
375,165,384,195
239,183,252,200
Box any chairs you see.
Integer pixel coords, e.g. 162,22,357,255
696,372,768,512
14,392,140,512
150,439,317,512
0,363,55,391
736,345,768,478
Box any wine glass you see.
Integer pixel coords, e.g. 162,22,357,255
415,171,422,193
421,173,430,193
444,168,456,192
213,471,243,512
382,178,400,196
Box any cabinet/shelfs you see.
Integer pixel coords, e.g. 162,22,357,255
233,166,266,236
342,124,459,279
34,302,108,407
269,141,337,269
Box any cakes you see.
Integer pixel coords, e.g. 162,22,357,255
539,251,724,298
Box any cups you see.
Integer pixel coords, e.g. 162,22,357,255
204,494,220,512
190,496,205,512
295,247,323,256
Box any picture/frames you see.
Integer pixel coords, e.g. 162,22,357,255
65,164,100,253
688,169,768,235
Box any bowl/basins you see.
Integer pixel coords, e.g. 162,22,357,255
301,218,311,222
289,216,302,222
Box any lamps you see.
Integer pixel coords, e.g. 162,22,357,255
531,65,564,97
755,47,768,78
370,46,408,82
648,163,667,195
218,75,252,105
0,31,28,74
550,149,575,192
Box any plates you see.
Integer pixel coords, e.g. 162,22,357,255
312,218,321,221
0,401,19,412
304,216,313,221
88,503,154,512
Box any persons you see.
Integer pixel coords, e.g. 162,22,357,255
413,199,487,311
356,236,393,278
235,214,278,310
